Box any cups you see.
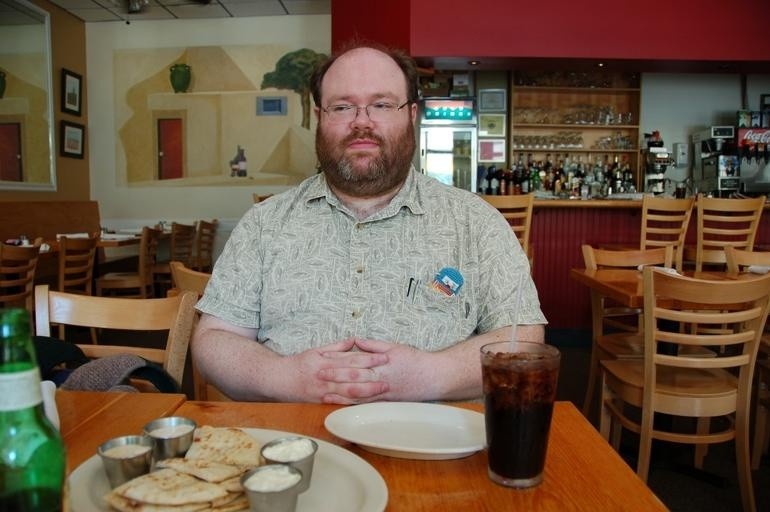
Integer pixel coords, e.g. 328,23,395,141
676,183,687,199
97,435,156,489
260,436,318,493
239,464,303,511
480,340,560,490
143,417,197,459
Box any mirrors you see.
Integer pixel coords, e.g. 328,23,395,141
0,1,57,191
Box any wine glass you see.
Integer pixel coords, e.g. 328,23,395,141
514,131,584,149
514,104,633,124
596,132,636,149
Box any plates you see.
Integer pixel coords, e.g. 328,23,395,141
325,402,486,460
66,426,388,511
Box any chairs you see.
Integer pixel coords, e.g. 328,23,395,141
0,219,219,394
478,191,534,252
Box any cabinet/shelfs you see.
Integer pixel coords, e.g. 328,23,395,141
513,77,638,171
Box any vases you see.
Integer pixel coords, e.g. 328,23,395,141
169,63,193,94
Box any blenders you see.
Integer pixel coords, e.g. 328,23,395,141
643,129,670,194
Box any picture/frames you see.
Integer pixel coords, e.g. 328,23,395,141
255,97,287,117
61,121,85,157
60,67,80,115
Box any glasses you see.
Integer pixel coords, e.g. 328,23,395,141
321,101,408,124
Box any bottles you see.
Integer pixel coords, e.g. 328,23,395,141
478,153,635,200
0,307,65,511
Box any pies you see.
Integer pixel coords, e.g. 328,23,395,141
103,425,260,512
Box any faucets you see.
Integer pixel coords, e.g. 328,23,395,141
662,177,672,192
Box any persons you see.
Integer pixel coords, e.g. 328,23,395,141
192,46,549,406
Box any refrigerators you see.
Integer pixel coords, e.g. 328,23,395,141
419,113,477,195
